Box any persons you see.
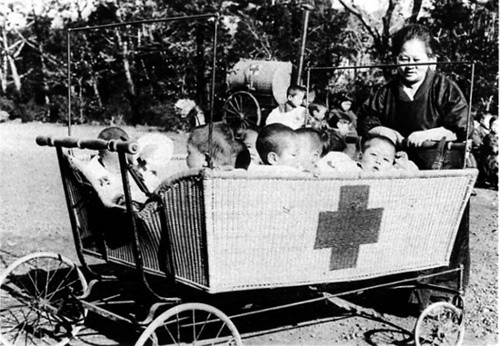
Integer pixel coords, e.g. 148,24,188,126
309,130,330,165
304,99,326,128
297,84,319,107
254,121,304,179
478,112,498,188
356,134,422,176
326,89,360,130
358,28,480,316
121,133,175,209
232,128,265,172
87,124,126,200
225,139,258,173
275,86,311,127
322,110,354,138
181,123,238,171
287,124,335,179
471,110,495,163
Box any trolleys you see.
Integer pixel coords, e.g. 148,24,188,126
1,125,481,346
